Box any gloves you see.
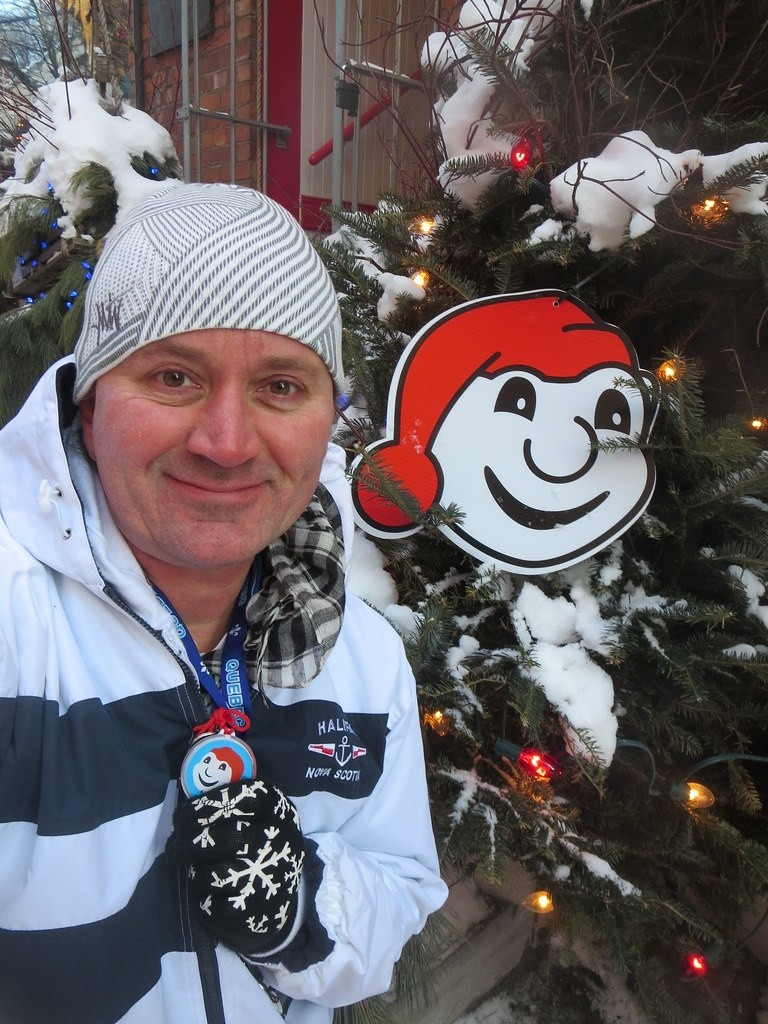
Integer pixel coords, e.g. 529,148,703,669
163,776,308,959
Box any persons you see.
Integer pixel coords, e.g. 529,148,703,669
0,183,448,1024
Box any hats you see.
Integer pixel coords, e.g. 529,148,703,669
71,182,346,406
